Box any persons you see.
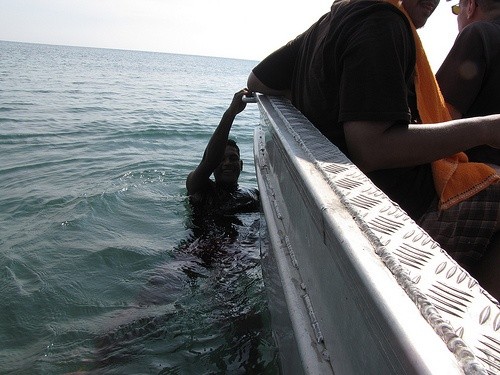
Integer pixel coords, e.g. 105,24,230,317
434,1,500,167
248,1,500,269
186,88,262,214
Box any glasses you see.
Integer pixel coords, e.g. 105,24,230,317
451,1,466,15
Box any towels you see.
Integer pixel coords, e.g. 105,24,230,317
386,0,500,215
